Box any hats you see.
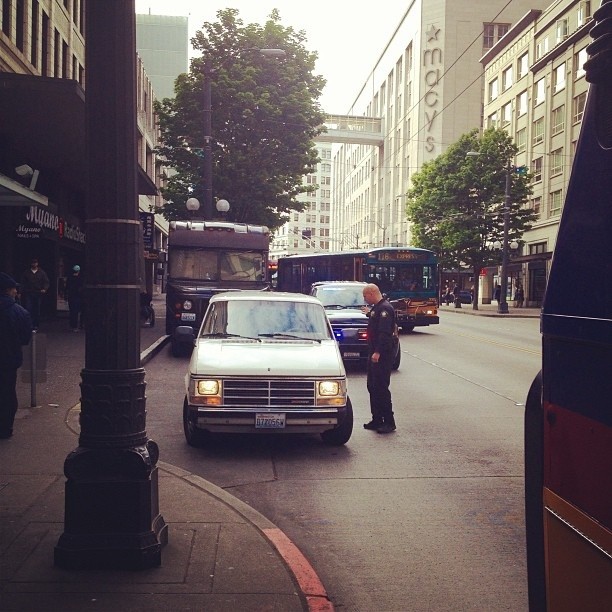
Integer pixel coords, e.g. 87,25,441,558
71,265,80,272
0,272,21,286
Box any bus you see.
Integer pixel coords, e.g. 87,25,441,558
158,220,273,357
277,247,439,331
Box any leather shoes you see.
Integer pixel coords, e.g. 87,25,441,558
378,423,396,433
363,421,383,429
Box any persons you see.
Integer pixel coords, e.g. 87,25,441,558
17,257,50,318
408,278,420,290
494,286,501,303
1,271,31,440
443,284,451,307
64,264,84,333
361,283,398,434
517,284,524,308
453,283,461,304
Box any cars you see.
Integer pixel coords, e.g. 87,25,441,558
309,281,401,369
183,290,353,447
